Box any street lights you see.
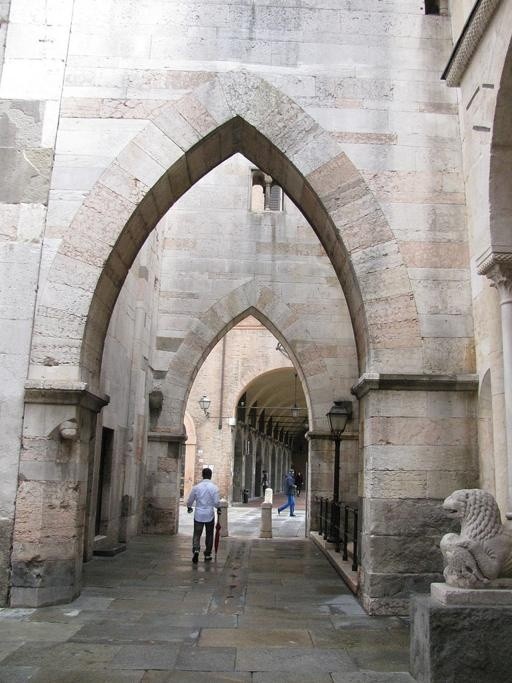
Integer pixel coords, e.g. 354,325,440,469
326,400,351,543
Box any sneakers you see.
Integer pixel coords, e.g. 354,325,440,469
290,513,296,516
278,507,280,515
192,550,199,563
204,555,213,560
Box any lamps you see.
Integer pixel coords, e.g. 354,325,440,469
198,394,211,417
302,416,309,431
290,372,300,418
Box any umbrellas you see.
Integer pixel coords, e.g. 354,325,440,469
214,510,222,557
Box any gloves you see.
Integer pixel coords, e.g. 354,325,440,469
217,508,221,515
187,507,193,513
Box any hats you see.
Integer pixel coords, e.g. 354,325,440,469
289,469,294,474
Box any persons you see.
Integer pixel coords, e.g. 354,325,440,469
278,469,298,517
294,472,304,497
184,468,221,565
261,469,269,497
